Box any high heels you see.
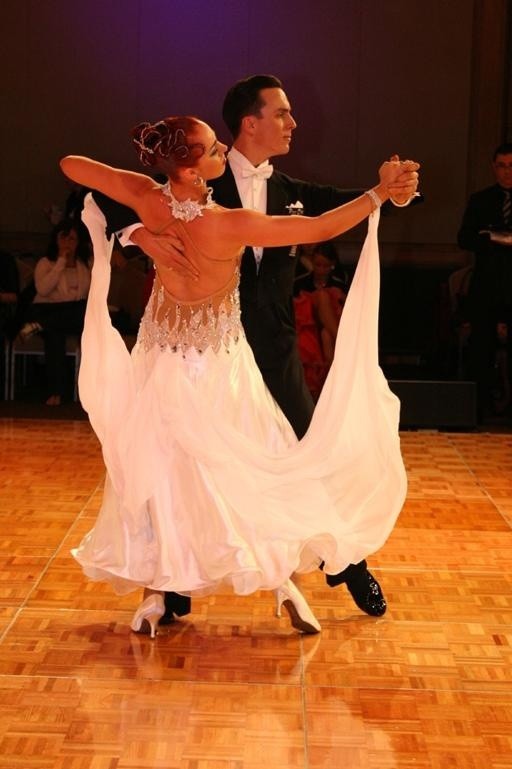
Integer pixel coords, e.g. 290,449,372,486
130,593,168,640
274,576,322,636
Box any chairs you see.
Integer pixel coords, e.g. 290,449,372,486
0,326,83,405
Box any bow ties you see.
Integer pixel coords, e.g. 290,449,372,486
238,161,276,196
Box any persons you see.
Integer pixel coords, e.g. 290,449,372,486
58,110,422,638
453,128,512,428
91,72,422,629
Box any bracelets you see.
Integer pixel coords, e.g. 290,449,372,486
364,186,384,211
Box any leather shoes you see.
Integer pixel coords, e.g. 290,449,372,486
345,567,387,617
157,608,179,625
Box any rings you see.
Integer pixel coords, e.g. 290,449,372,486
168,266,174,272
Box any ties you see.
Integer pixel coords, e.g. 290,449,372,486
503,191,511,231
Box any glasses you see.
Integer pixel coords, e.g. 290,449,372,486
492,162,511,171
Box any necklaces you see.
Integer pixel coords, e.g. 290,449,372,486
158,178,221,223
